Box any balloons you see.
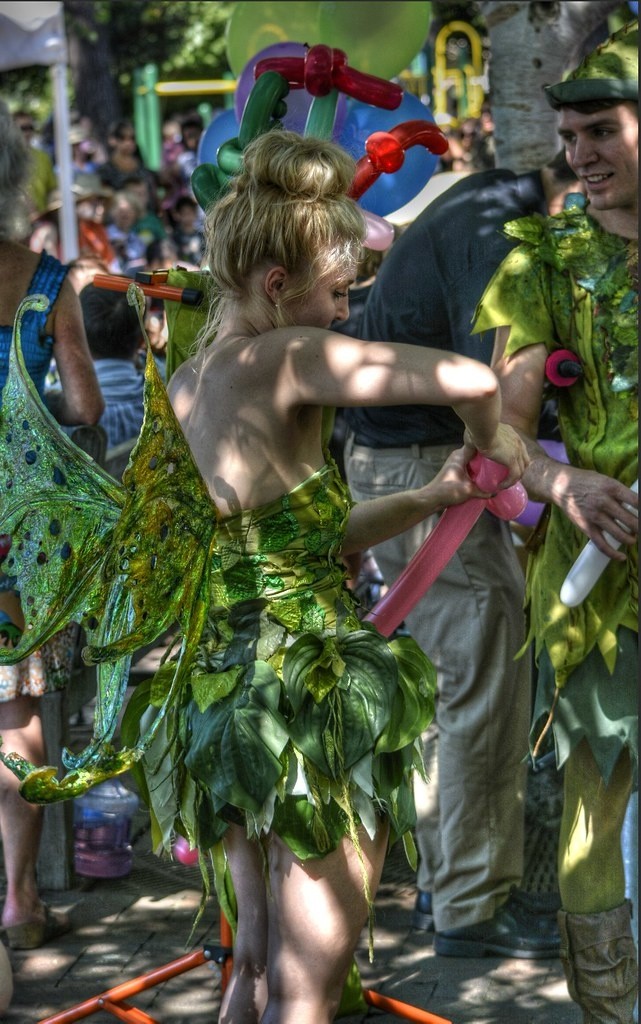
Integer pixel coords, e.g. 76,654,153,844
363,440,640,639
191,0,449,250
177,835,199,864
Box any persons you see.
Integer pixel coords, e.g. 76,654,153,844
2,117,584,960
1,130,529,1024
470,20,641,1024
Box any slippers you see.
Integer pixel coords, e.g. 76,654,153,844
5,901,70,949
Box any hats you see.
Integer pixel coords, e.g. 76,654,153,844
544,20,639,109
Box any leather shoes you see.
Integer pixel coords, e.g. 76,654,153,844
412,884,562,928
433,909,565,958
